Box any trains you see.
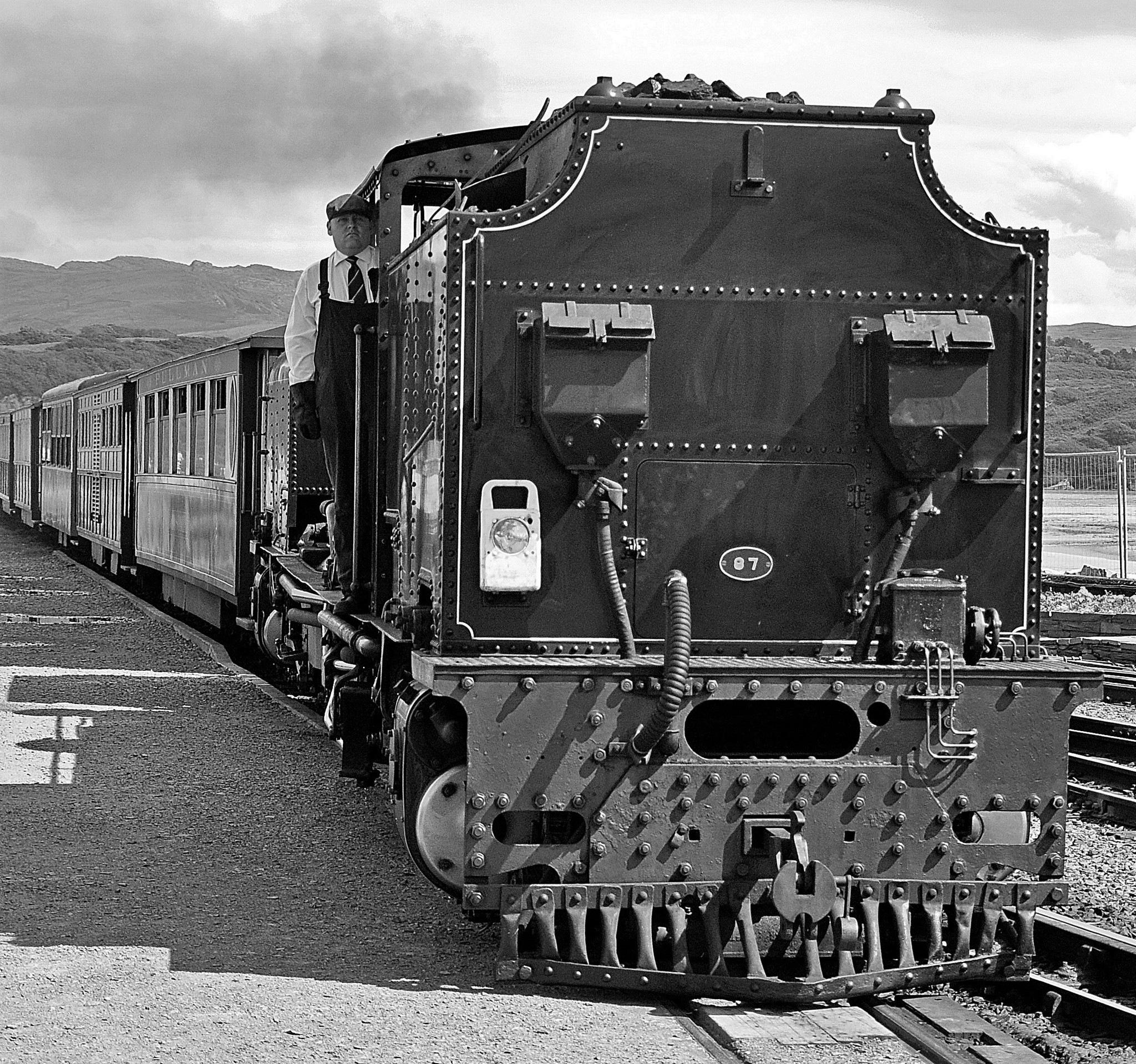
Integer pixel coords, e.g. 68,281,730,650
0,73,1107,1005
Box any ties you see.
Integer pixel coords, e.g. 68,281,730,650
345,256,368,305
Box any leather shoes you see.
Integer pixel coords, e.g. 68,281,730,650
332,596,370,615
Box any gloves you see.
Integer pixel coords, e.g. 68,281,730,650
290,381,321,440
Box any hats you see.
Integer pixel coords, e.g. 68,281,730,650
326,194,372,222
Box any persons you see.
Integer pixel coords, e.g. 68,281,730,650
283,194,380,614
